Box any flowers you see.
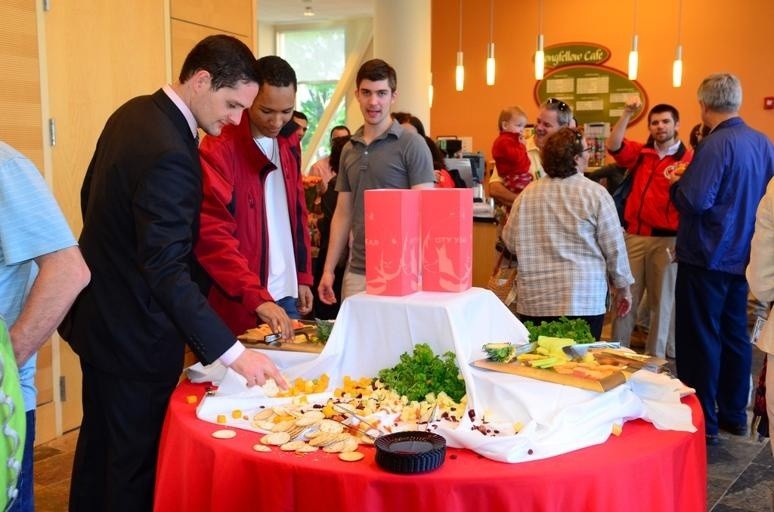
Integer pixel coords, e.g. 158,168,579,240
300,172,323,247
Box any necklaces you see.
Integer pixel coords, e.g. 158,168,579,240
252,136,274,166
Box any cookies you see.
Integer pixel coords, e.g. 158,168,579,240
252,404,364,460
212,429,237,439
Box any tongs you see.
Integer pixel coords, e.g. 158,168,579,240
264,326,313,346
562,341,621,360
593,351,664,374
324,403,383,440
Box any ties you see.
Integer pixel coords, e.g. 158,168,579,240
194,132,199,145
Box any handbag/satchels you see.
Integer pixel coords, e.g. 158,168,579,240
486,245,517,316
587,163,633,224
753,352,769,438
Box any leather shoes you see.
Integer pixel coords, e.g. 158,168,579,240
718,418,748,435
705,433,719,444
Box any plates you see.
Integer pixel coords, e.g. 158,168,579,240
374,431,447,473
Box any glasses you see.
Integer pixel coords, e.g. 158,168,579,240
547,98,567,111
582,145,592,153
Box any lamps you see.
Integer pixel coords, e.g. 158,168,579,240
534,0,547,82
671,0,686,89
454,0,466,93
427,70,435,109
484,0,498,87
626,0,642,82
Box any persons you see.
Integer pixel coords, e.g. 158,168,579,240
59,34,312,512
1,140,90,511
304,59,772,461
